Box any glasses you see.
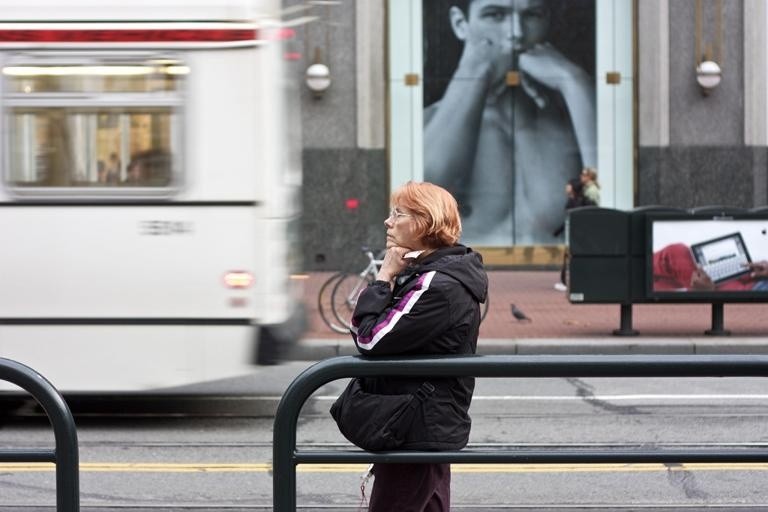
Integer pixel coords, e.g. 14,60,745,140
391,208,413,220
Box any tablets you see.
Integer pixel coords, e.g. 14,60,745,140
689,232,754,284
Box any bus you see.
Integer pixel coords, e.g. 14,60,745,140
0,0,288,393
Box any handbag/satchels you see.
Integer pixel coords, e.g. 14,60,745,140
331,377,420,452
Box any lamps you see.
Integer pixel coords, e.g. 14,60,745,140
695,53,723,97
306,47,331,101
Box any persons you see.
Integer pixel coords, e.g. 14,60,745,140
580,166,601,204
330,182,489,511
653,243,768,291
424,1,596,245
551,180,597,292
